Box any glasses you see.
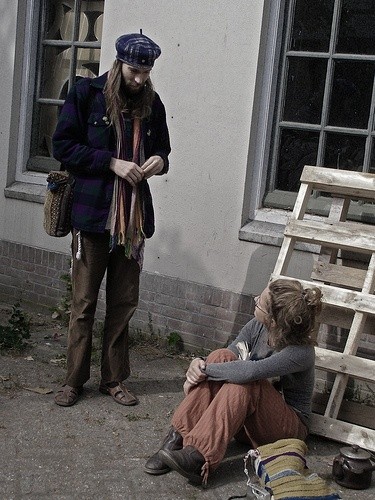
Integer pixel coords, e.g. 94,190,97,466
253,295,268,316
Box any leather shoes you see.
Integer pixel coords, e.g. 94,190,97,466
159,445,207,483
142,426,184,475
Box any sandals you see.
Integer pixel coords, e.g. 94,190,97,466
107,381,137,406
54,384,84,406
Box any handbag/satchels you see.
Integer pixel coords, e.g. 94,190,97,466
243,437,340,500
41,167,74,238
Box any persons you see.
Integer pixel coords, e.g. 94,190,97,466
52,29,172,405
143,279,323,489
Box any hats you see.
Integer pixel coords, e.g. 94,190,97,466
115,28,162,69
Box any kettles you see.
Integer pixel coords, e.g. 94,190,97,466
333,445,372,490
